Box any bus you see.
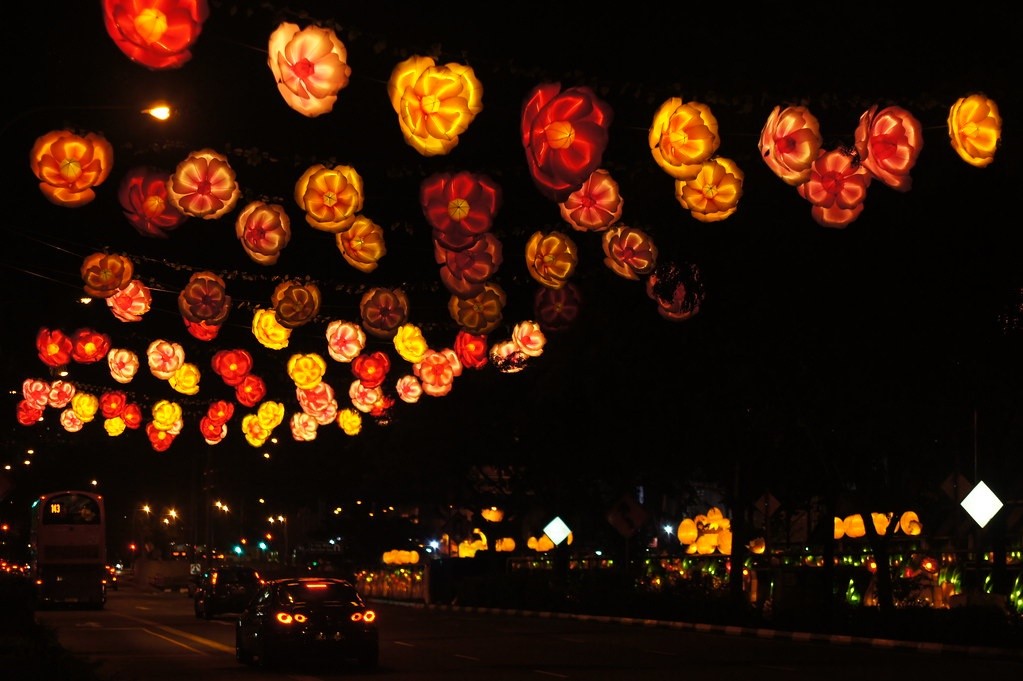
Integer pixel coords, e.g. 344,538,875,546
29,490,109,611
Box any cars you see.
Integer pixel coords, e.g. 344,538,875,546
193,563,266,620
234,576,379,672
